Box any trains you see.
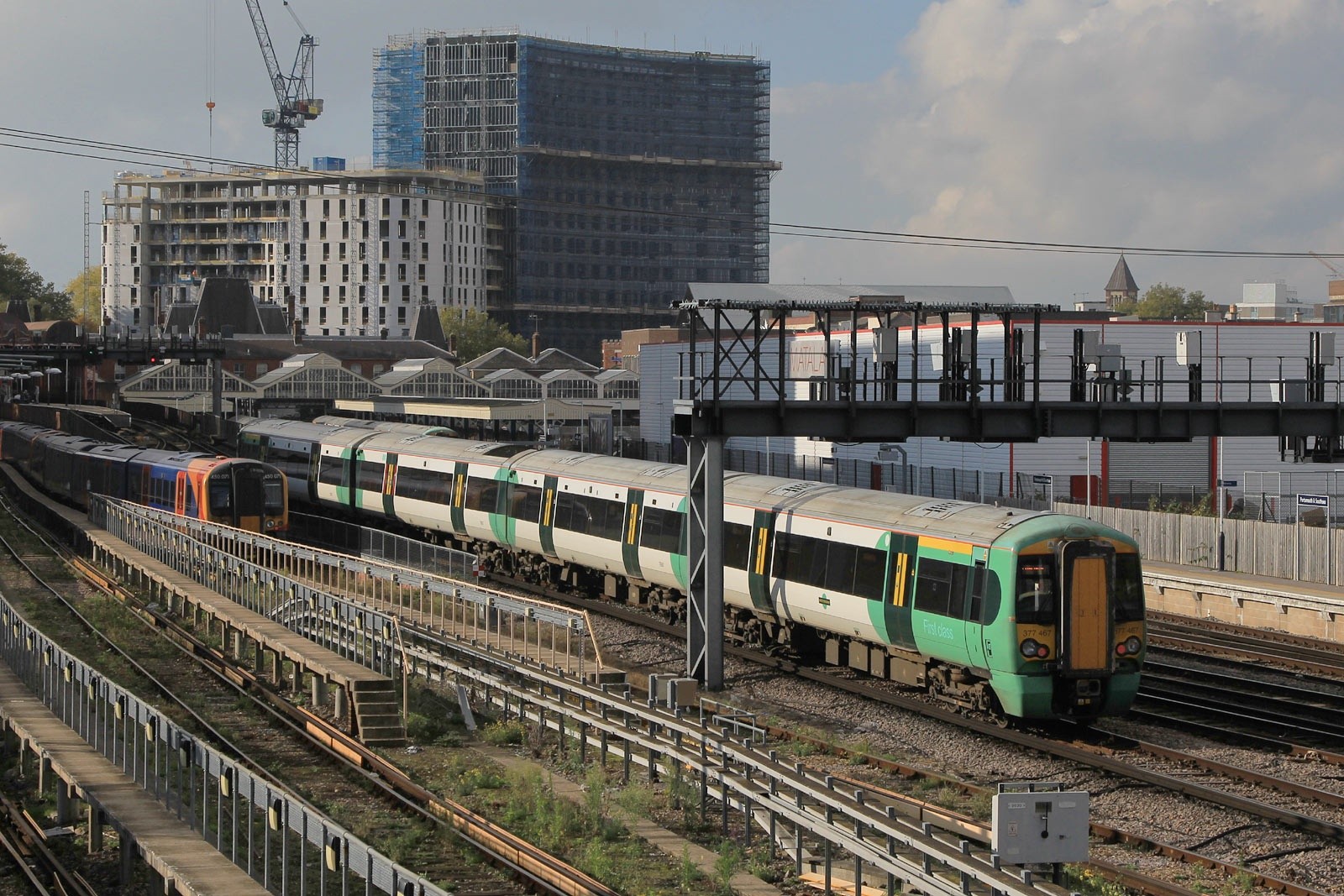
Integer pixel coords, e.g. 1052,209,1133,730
0,420,288,538
236,417,1145,729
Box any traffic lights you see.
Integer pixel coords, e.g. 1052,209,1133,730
87,344,96,360
146,352,159,365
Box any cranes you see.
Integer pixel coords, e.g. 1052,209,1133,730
1309,250,1344,277
244,0,324,171
